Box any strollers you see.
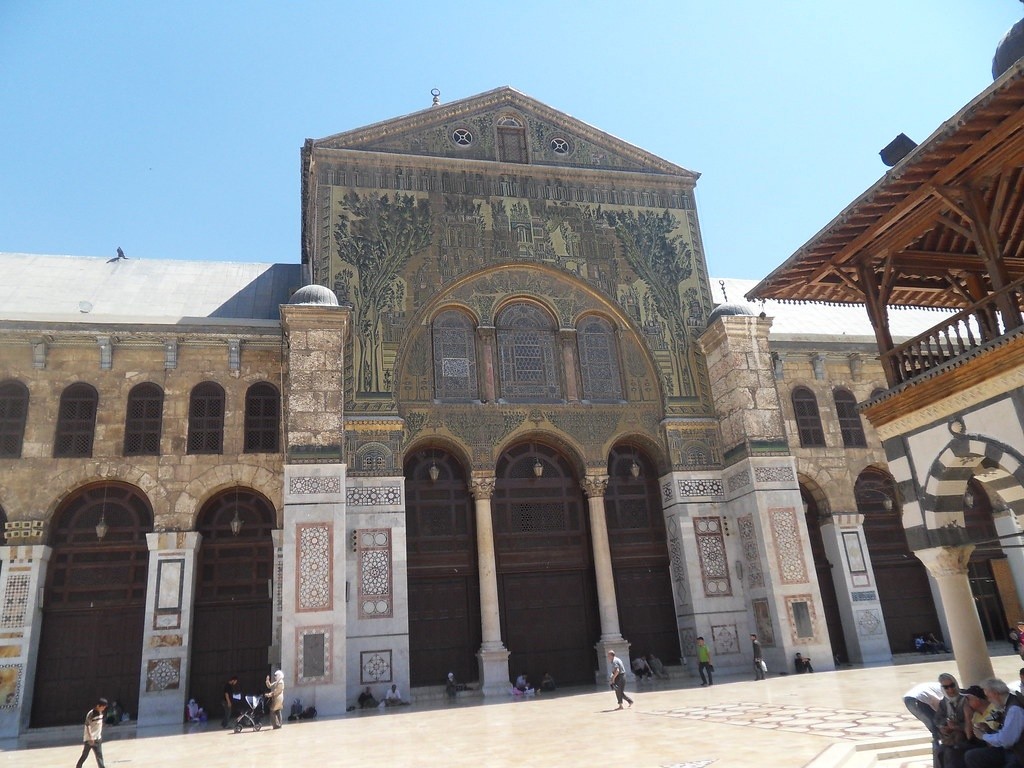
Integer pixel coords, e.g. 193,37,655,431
233,695,272,733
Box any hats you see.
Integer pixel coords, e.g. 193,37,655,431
697,637,704,641
960,685,987,701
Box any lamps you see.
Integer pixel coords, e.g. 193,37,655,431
231,487,243,535
883,494,893,511
429,446,439,485
532,438,544,479
95,483,109,543
966,493,974,509
630,445,640,480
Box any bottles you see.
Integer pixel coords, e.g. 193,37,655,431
612,684,619,690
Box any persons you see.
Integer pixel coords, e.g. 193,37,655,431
185,699,202,721
385,684,400,706
632,654,668,681
291,698,302,719
516,672,530,690
76,697,108,768
915,633,951,654
608,650,633,710
265,670,284,729
358,687,376,708
105,700,121,725
795,653,813,673
1008,622,1024,651
538,672,555,692
221,676,238,729
933,668,1024,768
750,633,765,681
696,637,713,685
446,672,457,696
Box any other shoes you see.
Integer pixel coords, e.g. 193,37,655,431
709,683,713,685
700,681,707,686
755,677,760,681
762,677,766,680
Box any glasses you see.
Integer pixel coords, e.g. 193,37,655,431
942,683,956,690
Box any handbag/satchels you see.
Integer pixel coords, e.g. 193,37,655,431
760,660,768,671
709,664,714,672
